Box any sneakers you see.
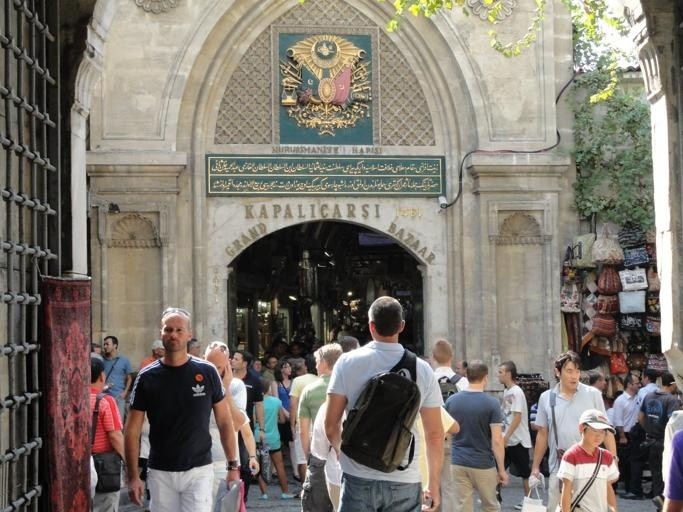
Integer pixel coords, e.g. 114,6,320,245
258,494,268,498
653,495,664,508
281,492,295,499
514,502,523,509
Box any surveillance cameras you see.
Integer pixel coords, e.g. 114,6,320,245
438,196,449,210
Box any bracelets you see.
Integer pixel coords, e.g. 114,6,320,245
225,466,240,470
247,455,255,459
226,459,240,466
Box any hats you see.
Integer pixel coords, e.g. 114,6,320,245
579,410,617,435
152,339,165,351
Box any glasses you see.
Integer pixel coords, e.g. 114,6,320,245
585,426,608,435
209,343,229,363
496,483,503,503
159,307,190,319
634,381,640,386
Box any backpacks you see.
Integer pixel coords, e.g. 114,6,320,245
437,374,462,402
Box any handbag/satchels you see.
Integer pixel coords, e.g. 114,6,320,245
609,354,628,375
598,265,621,295
592,225,625,265
580,346,608,370
626,352,646,369
620,316,643,331
598,295,619,314
521,496,548,512
606,375,625,398
256,447,272,485
589,336,612,355
646,317,661,336
571,233,596,268
618,292,646,314
647,294,662,315
647,355,670,377
629,333,647,352
648,268,661,292
619,266,647,292
93,450,121,492
618,222,647,249
592,316,616,337
623,248,649,267
647,245,657,264
613,340,627,353
341,349,420,474
629,368,642,380
560,282,581,314
614,440,631,457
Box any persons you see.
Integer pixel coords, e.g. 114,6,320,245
298,343,343,512
415,406,460,512
248,358,263,376
89,406,96,415
231,350,265,508
205,341,246,512
139,340,165,371
236,341,268,360
579,354,622,409
530,350,608,512
208,406,259,512
621,369,660,500
187,338,201,358
125,308,241,512
662,429,682,512
262,356,278,382
529,402,543,432
310,401,346,512
326,315,373,352
661,409,682,486
432,338,469,404
405,343,431,366
270,361,300,482
286,342,306,372
89,343,103,361
294,363,307,376
324,296,442,512
253,375,296,499
272,341,289,365
444,360,508,512
638,374,682,498
454,360,467,377
99,336,132,421
89,357,125,512
497,361,532,509
556,408,620,512
612,374,642,494
289,355,318,500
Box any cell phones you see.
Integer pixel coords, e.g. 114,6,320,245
103,382,113,392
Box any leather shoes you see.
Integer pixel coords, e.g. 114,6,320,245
620,493,643,500
